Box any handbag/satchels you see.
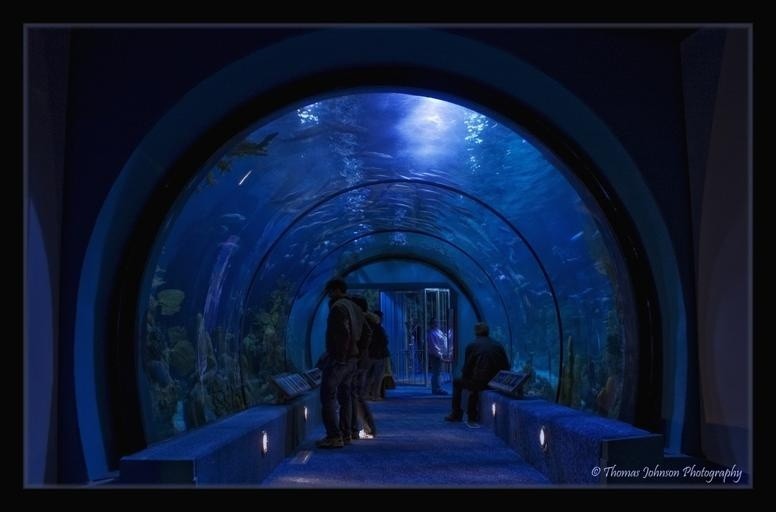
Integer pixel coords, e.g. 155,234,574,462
384,374,396,390
318,352,336,372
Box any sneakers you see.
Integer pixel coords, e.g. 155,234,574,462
444,409,463,422
316,429,374,448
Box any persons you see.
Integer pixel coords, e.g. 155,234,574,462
313,276,391,448
427,316,449,395
444,320,510,423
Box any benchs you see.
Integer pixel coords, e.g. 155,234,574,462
266,385,322,450
120,404,295,488
460,386,543,434
515,398,664,489
